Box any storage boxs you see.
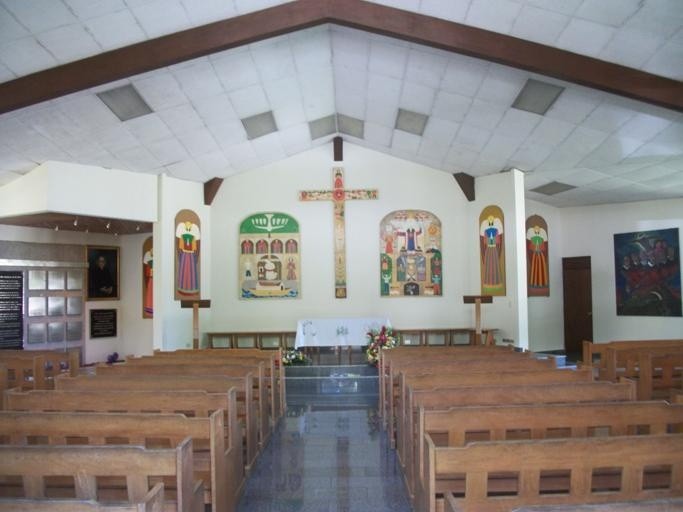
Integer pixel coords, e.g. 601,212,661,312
205,327,482,365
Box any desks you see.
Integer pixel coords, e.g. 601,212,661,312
294,317,396,363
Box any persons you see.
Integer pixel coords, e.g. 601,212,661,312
92,255,116,296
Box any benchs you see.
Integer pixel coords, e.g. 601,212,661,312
376,338,682,512
1,347,287,512
205,327,482,365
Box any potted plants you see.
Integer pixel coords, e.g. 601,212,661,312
83,244,121,302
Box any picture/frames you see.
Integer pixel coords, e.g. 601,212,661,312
83,244,121,302
294,317,396,363
